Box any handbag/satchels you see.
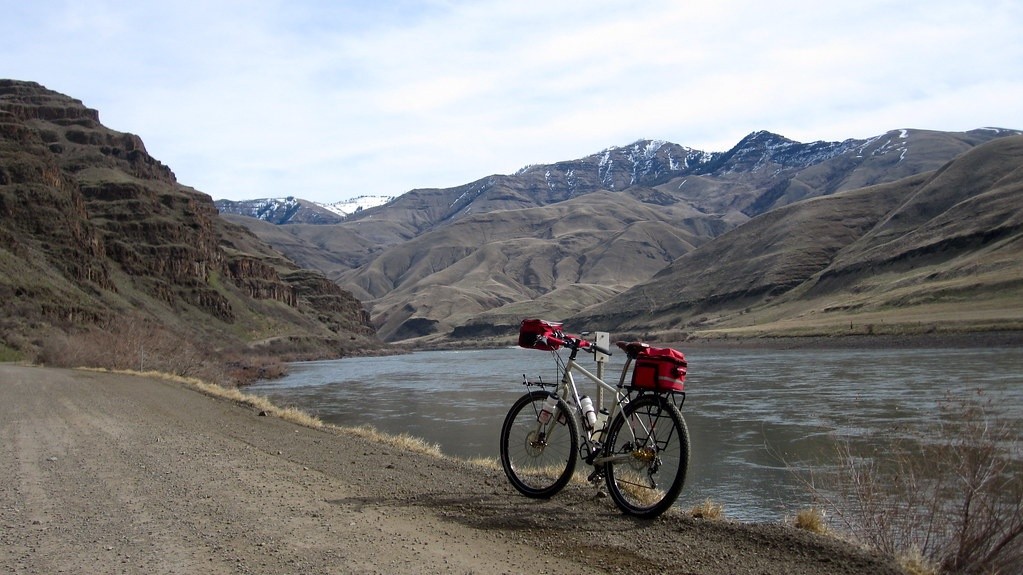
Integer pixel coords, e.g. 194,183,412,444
518,317,564,352
631,346,687,390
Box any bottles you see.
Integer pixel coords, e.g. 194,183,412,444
578,394,598,427
591,407,610,443
537,391,558,425
557,392,583,426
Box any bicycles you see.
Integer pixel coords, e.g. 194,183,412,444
498,331,692,518
